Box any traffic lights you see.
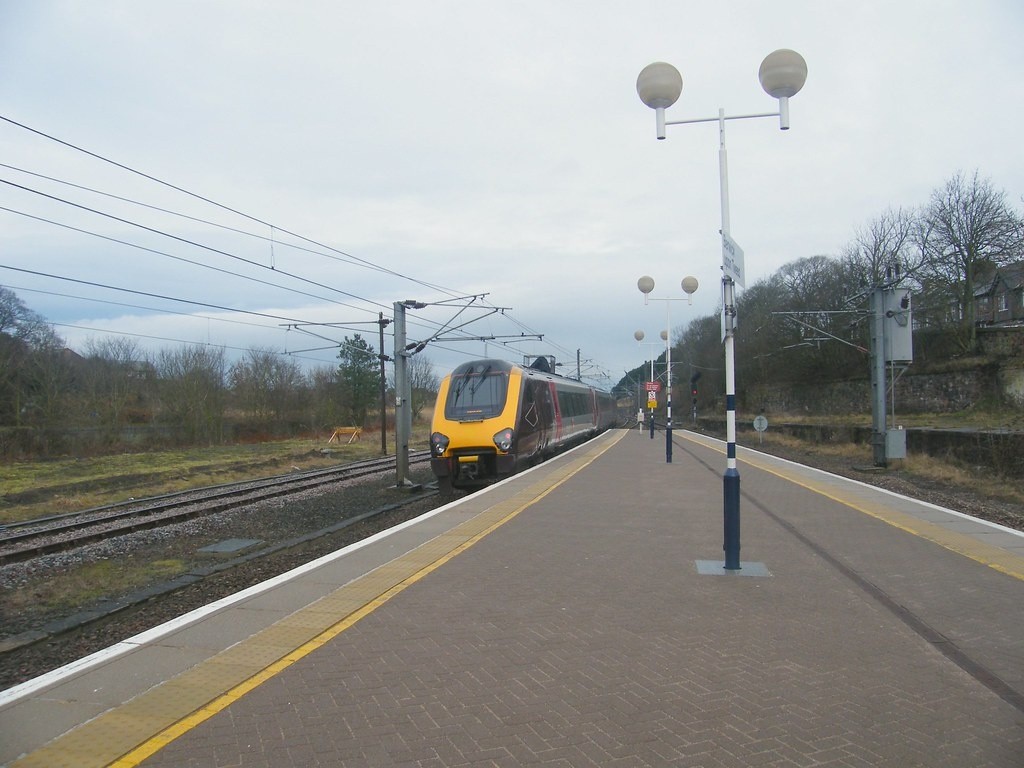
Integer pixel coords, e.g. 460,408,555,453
691,384,698,397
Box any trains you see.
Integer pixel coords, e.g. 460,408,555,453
430,355,617,496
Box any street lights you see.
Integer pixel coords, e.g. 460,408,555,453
634,329,667,439
635,49,808,570
638,275,698,462
